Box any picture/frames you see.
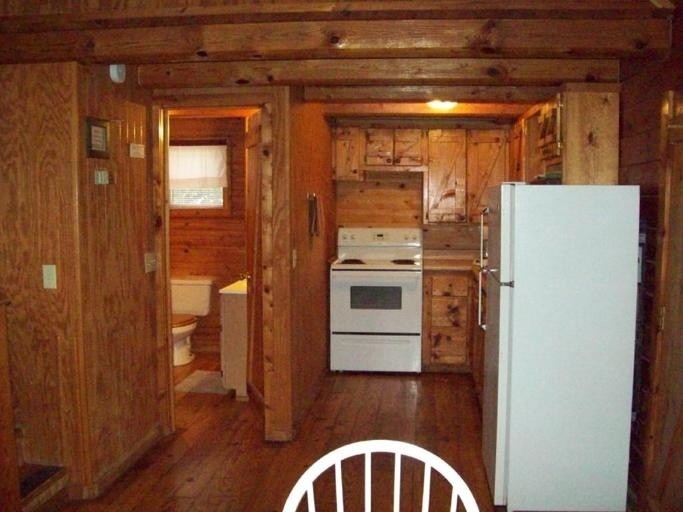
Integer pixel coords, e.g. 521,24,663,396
87,116,110,159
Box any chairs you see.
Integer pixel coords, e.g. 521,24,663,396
282,440,481,511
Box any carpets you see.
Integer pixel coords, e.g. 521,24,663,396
175,369,230,394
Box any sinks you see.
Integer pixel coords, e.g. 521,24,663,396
220,278,248,295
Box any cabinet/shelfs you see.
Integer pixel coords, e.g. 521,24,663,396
425,121,510,227
510,91,613,202
328,123,424,182
424,247,486,406
219,294,250,401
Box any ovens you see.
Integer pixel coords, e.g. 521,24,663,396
331,269,421,336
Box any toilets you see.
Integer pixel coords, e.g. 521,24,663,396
170,274,212,367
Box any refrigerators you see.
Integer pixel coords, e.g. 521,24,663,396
477,182,639,507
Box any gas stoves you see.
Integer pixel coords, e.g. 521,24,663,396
331,227,422,265
